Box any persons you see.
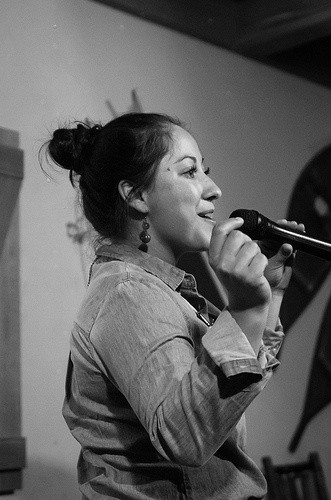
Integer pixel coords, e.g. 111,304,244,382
36,112,305,500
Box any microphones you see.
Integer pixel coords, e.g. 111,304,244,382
228,208,331,262
